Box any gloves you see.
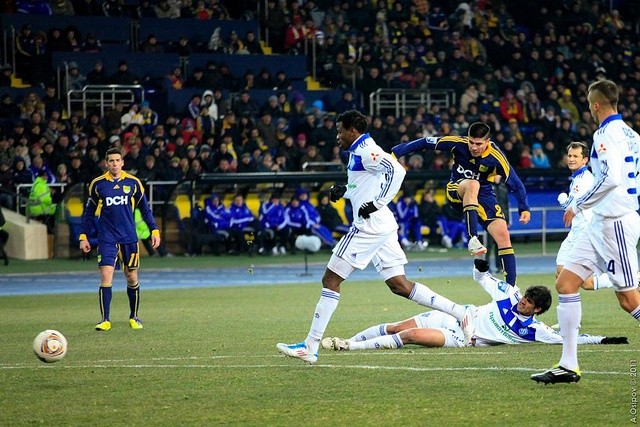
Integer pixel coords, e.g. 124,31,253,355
601,337,628,344
358,201,377,219
328,185,347,203
475,259,490,272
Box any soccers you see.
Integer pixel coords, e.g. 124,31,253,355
33,329,68,363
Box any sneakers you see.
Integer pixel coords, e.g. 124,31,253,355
441,235,453,248
276,343,318,364
332,338,350,351
530,365,581,385
129,315,143,330
322,337,344,349
467,236,487,256
458,305,477,347
95,320,111,331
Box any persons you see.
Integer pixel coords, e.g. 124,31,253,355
204,193,236,256
315,190,350,235
79,146,162,331
441,200,469,249
229,194,259,255
418,192,453,250
321,257,630,352
0,0,121,235
203,0,469,172
259,191,289,257
285,195,314,256
556,142,613,292
121,0,203,217
276,109,478,365
390,121,533,289
387,200,411,247
529,79,639,384
396,194,428,252
295,187,339,250
468,0,639,169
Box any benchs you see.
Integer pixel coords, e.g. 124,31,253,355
476,191,573,241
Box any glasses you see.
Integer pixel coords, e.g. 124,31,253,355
16,125,23,128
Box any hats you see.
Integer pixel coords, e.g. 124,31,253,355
194,67,203,72
297,133,306,140
94,59,102,64
578,123,591,134
37,169,44,176
312,100,323,111
206,61,217,67
184,145,197,151
561,109,572,116
119,61,126,67
87,32,96,40
376,12,384,18
562,89,572,95
109,135,121,143
294,91,305,101
0,160,9,166
324,115,336,122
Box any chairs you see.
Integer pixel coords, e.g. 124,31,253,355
174,187,473,257
65,196,104,261
